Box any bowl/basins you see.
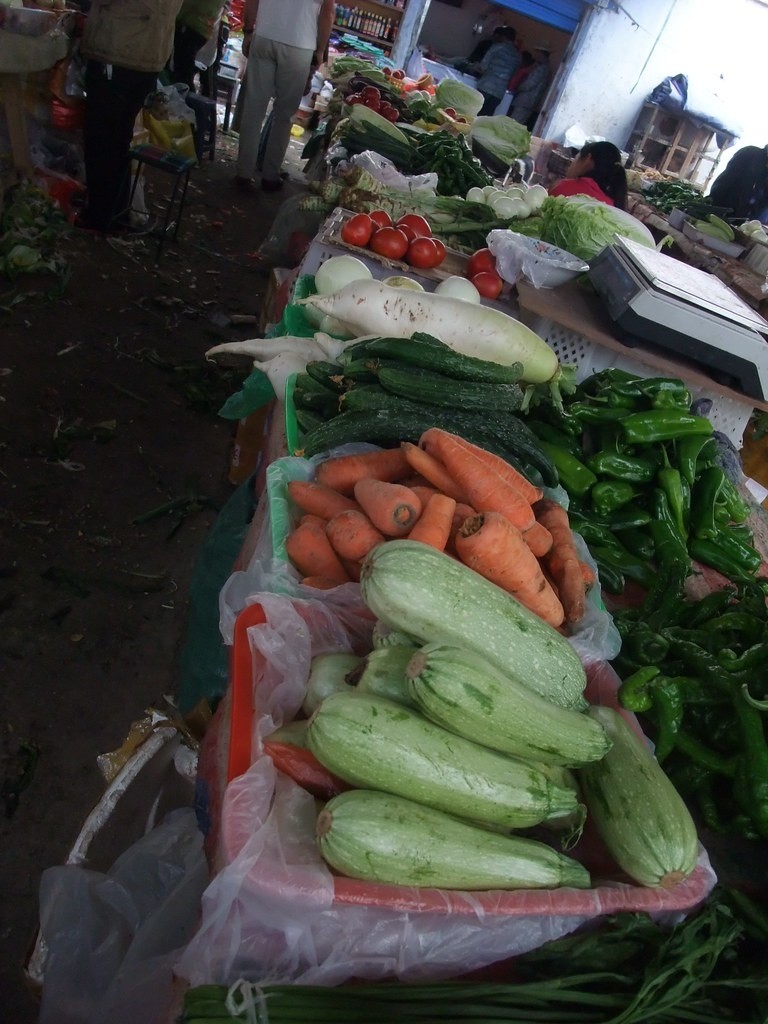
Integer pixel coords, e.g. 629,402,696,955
505,233,591,290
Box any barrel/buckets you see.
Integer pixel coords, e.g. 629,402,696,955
301,86,321,108
293,105,316,127
35,164,85,227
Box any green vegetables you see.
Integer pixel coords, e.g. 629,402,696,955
645,180,712,212
183,889,768,1024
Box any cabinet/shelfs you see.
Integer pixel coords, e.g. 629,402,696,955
332,0,411,59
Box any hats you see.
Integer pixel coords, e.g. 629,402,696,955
532,40,551,53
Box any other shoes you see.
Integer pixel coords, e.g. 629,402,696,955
78,211,129,231
261,177,284,193
237,173,257,192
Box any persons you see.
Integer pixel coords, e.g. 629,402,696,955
477,27,523,118
710,146,768,224
548,141,628,212
172,0,226,93
235,0,336,193
466,26,504,64
75,0,186,233
507,51,535,91
509,39,554,126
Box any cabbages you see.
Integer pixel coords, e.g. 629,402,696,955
435,78,484,116
471,115,532,164
512,190,672,266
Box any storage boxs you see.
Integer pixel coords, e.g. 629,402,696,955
285,371,312,459
525,305,754,448
34,165,91,223
668,207,694,231
266,456,611,626
682,219,747,258
299,234,520,320
199,600,715,961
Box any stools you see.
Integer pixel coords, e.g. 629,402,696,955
102,143,197,262
217,73,237,134
184,90,217,162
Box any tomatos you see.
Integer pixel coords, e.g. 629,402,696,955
341,209,446,268
468,248,497,273
346,86,398,122
382,67,405,80
472,272,503,299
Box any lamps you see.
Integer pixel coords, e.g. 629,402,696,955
471,13,487,35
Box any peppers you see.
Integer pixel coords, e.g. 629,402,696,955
543,368,768,841
416,129,495,197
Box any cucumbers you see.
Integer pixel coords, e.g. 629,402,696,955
341,120,425,175
286,333,560,489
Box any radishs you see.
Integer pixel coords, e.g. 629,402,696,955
206,279,575,406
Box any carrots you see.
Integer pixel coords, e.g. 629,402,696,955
286,427,595,635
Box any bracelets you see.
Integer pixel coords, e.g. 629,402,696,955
242,28,254,34
